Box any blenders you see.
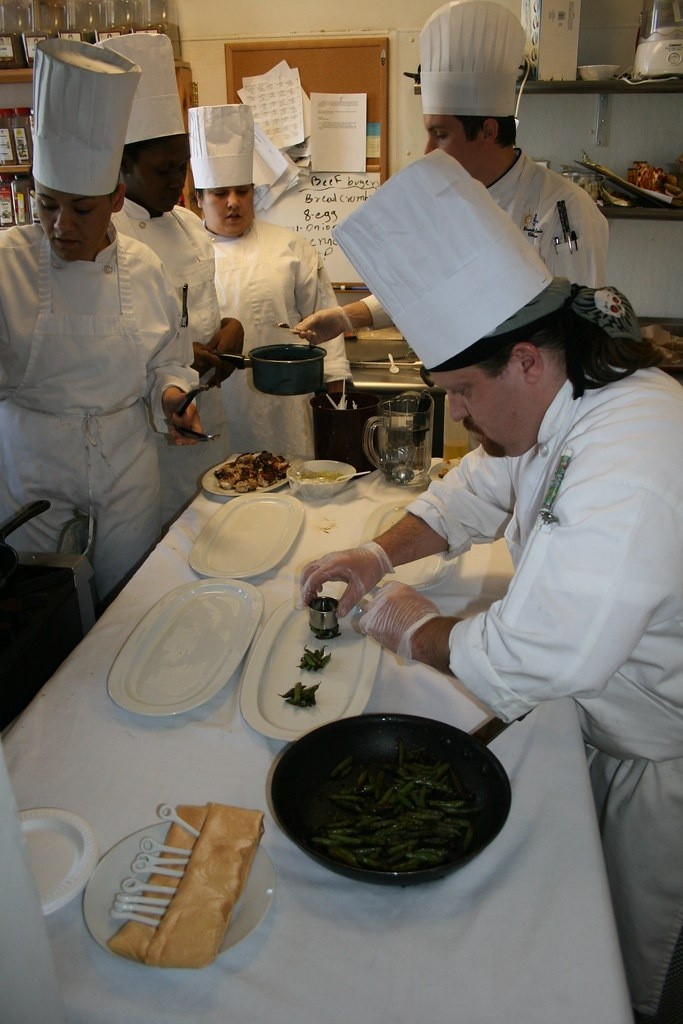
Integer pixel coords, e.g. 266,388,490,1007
630,1,683,80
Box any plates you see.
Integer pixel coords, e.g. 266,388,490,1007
83,821,274,961
239,589,381,740
201,454,303,496
361,499,460,593
107,578,263,716
18,808,99,916
428,457,461,481
188,494,305,578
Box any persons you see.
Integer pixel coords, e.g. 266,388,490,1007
188,104,353,461
93,32,244,538
294,0,609,346
298,148,683,1023
0,38,202,610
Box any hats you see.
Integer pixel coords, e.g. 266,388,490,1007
93,33,186,145
187,104,254,188
32,38,142,196
419,0,527,116
331,148,553,369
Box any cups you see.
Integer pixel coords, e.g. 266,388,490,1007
363,393,434,486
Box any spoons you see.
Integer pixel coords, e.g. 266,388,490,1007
387,352,400,374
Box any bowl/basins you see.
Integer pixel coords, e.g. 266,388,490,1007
287,461,355,498
575,63,621,80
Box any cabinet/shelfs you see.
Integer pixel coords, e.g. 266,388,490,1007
411,79,683,377
0,61,203,234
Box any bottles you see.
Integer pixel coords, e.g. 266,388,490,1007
0,107,18,165
11,107,33,165
0,173,40,228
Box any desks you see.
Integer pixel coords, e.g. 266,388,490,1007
1,458,643,1024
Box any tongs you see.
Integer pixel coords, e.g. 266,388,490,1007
171,382,222,443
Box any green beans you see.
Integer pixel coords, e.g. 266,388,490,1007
307,736,482,874
311,622,340,639
278,644,333,707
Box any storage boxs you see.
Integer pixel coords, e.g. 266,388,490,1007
520,0,581,81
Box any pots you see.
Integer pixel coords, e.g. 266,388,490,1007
266,706,532,884
0,500,52,589
214,343,328,395
